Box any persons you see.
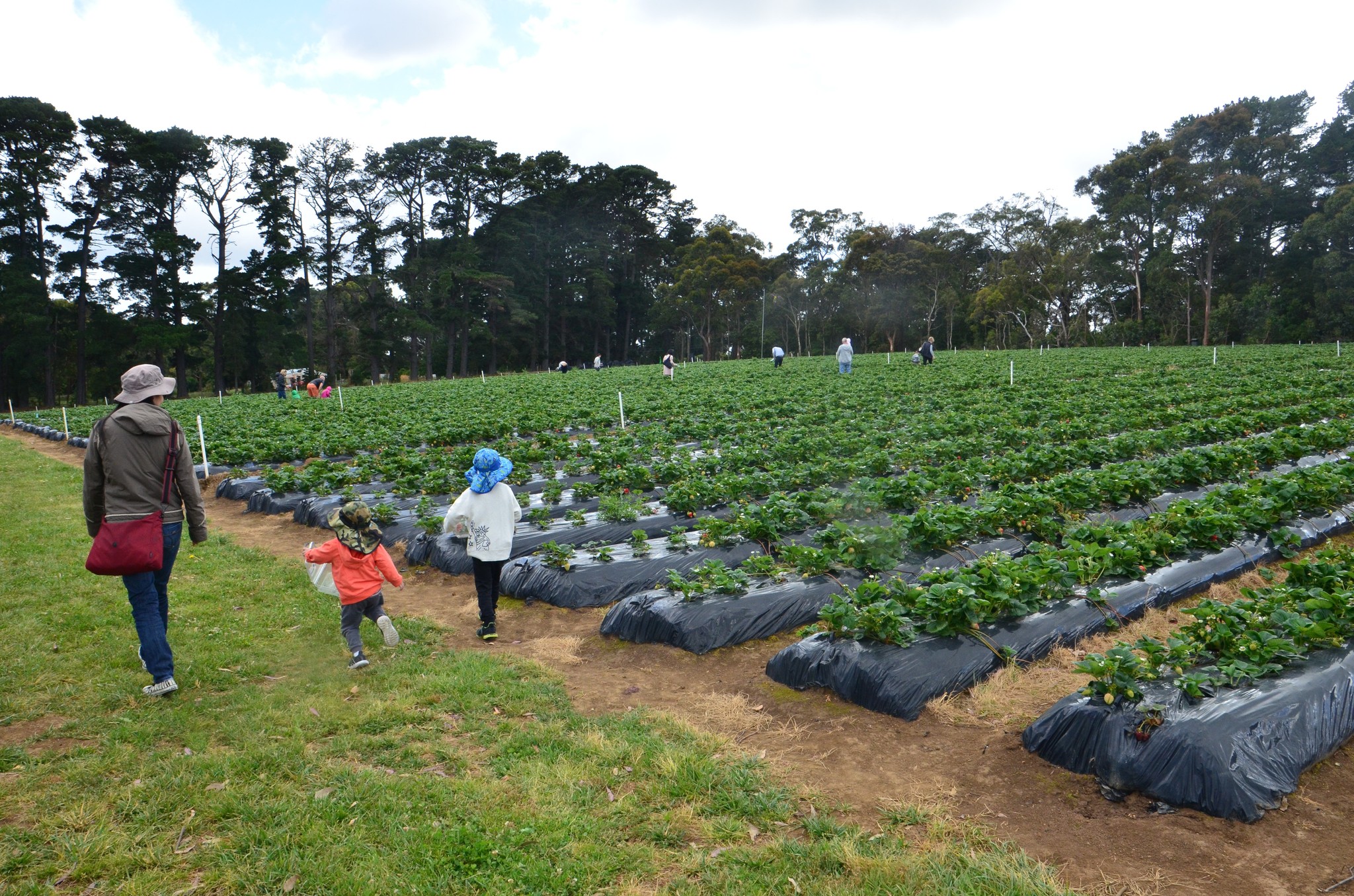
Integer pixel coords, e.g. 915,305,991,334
307,375,325,398
911,349,921,365
321,386,332,398
772,346,784,369
81,363,209,700
847,338,851,346
558,361,568,374
593,353,601,371
301,501,405,670
291,390,301,399
662,349,679,376
441,448,522,639
837,337,854,374
275,369,291,399
919,336,935,365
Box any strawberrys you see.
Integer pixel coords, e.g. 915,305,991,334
10,327,1354,744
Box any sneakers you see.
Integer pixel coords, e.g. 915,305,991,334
478,610,497,621
346,650,370,669
377,615,399,648
475,622,499,640
138,645,148,670
142,676,179,697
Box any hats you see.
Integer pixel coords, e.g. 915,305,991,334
915,349,919,353
113,364,176,405
327,501,384,555
463,447,514,494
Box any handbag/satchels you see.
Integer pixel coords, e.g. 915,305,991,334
918,346,923,354
662,358,672,370
83,508,164,576
